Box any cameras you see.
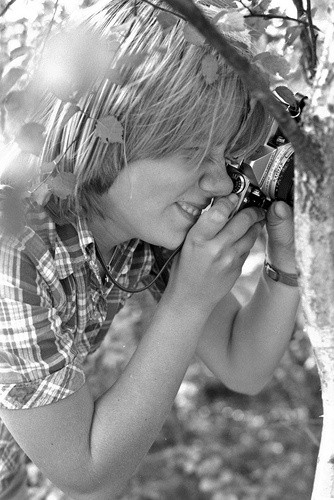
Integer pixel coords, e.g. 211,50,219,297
211,86,308,219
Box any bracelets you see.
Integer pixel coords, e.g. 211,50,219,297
262,256,301,288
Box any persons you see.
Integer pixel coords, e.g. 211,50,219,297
0,0,303,500
